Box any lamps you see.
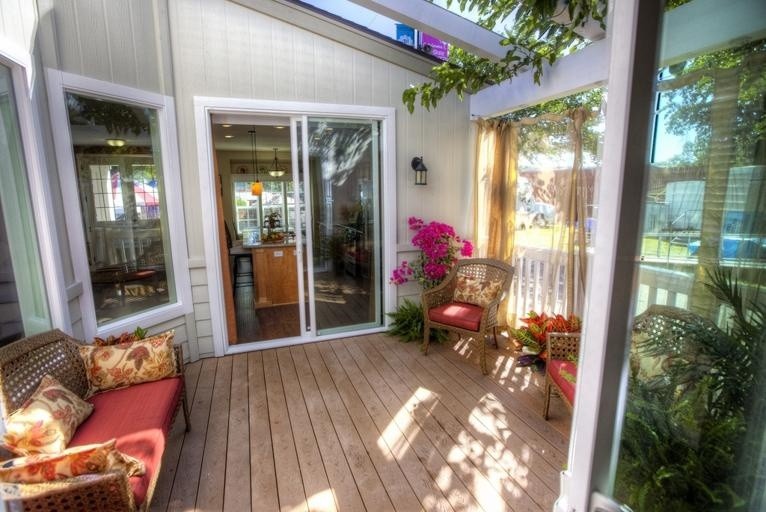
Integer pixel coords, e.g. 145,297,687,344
104,138,126,148
248,130,285,195
411,156,430,186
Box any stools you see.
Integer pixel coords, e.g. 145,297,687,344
232,253,254,297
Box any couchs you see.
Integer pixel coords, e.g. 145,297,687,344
0,328,191,512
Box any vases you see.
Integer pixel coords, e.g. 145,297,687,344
422,287,450,340
532,359,561,397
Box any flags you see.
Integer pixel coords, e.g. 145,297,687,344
395,22,414,49
419,30,447,61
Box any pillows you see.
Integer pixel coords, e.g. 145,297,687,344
453,276,504,307
0,438,146,483
78,330,177,400
0,373,93,454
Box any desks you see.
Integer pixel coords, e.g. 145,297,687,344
90,269,159,306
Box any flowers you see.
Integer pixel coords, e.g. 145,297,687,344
509,308,582,370
389,216,474,289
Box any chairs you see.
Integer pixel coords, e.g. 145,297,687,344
422,259,515,376
544,304,719,421
